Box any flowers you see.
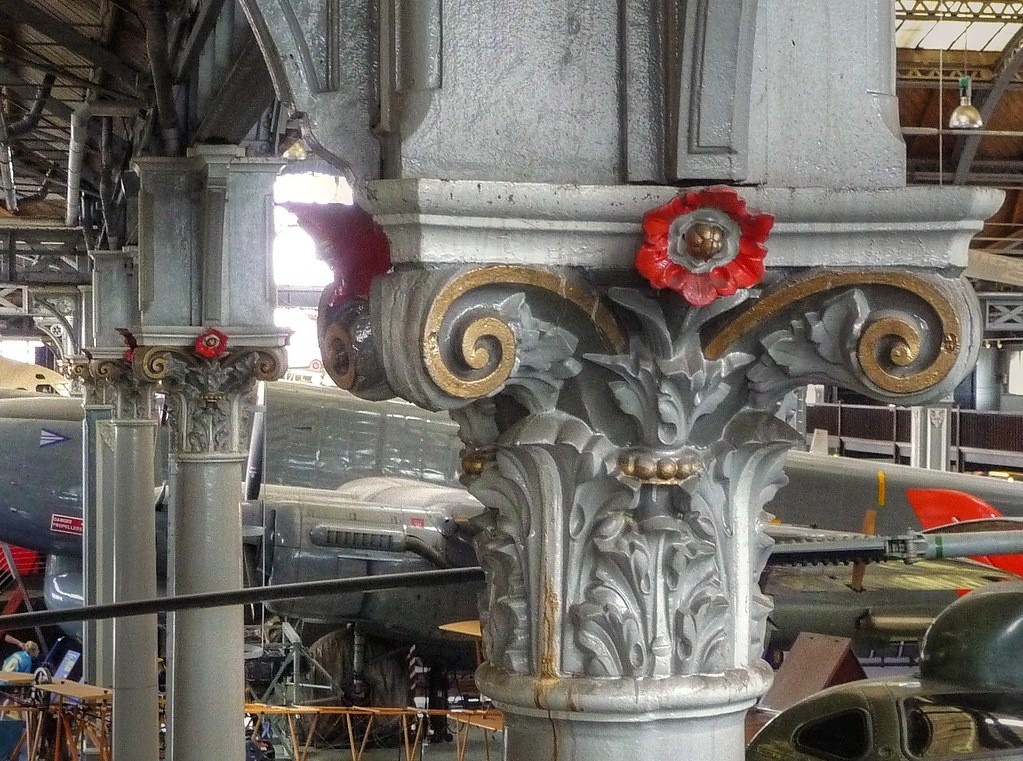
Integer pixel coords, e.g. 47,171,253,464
635,188,775,305
275,202,394,304
194,327,229,359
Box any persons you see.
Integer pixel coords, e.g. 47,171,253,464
0,640,38,720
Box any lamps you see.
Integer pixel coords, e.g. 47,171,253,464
278,132,308,164
948,2,983,128
